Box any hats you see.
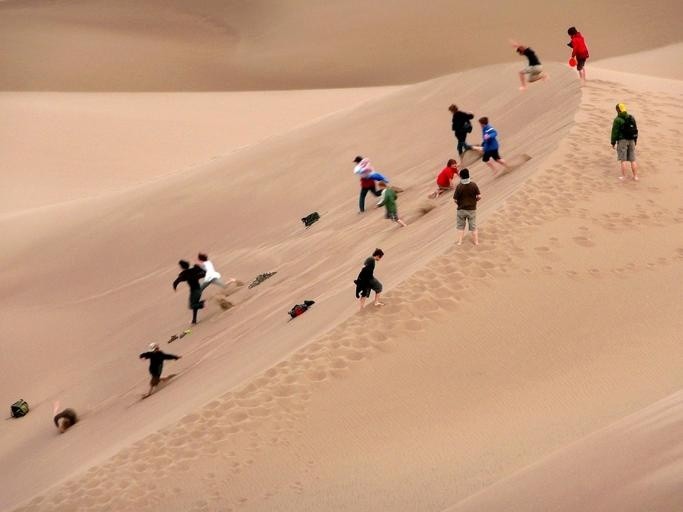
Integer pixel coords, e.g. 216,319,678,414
616,103,627,114
148,343,157,351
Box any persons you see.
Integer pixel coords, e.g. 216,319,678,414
568,27,589,84
139,343,182,398
354,250,384,309
353,156,382,212
611,102,639,181
197,254,236,292
479,117,510,176
172,261,206,324
513,43,547,91
453,169,481,245
448,105,483,168
375,182,405,227
53,401,76,433
429,159,460,198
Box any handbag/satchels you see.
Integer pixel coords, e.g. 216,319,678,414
10,399,29,417
